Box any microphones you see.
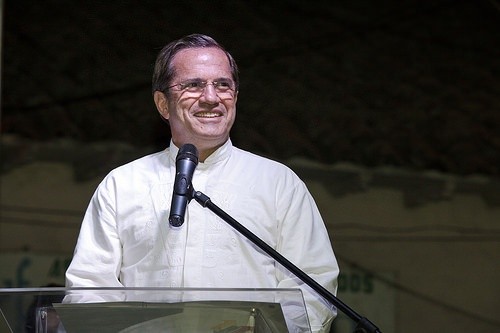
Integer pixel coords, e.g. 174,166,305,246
169,143,199,227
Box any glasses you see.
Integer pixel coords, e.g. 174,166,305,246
167,80,236,94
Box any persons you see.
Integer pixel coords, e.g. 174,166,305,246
62,33,340,333
